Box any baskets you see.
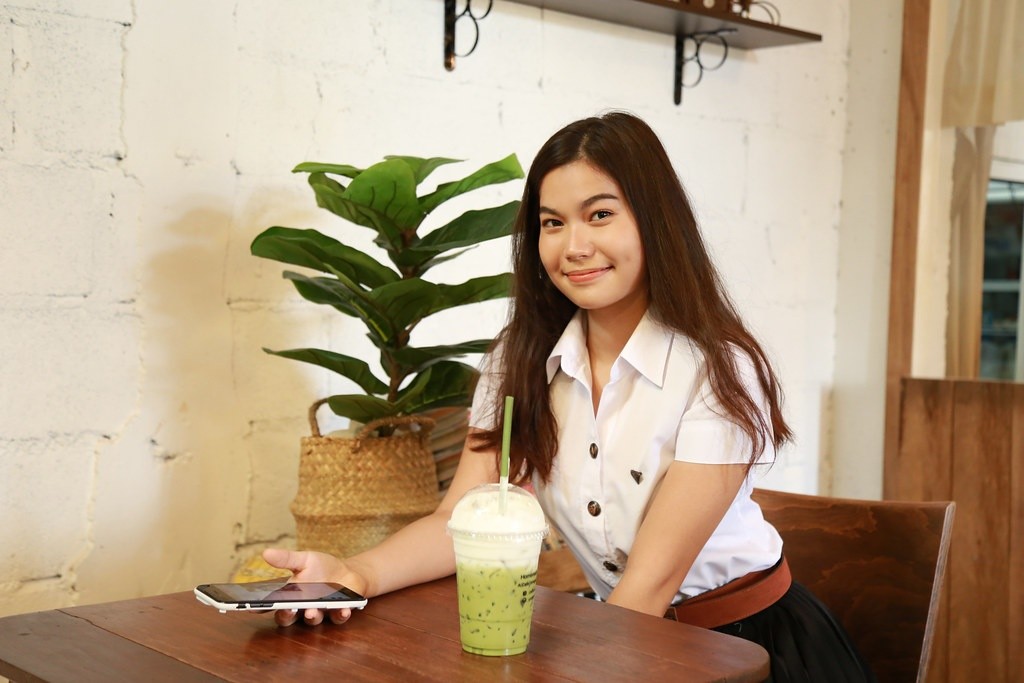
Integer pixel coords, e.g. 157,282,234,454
291,397,443,559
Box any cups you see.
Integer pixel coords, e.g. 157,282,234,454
448,482,550,657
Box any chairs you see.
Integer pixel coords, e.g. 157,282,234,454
750,486,957,683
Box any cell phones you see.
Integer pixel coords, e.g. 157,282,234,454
194,582,368,613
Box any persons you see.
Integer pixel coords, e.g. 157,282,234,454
249,110,866,683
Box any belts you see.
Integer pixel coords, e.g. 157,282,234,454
664,554,791,629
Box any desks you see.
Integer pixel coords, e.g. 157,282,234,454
0,570,772,683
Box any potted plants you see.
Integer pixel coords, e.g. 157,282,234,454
251,154,525,564
